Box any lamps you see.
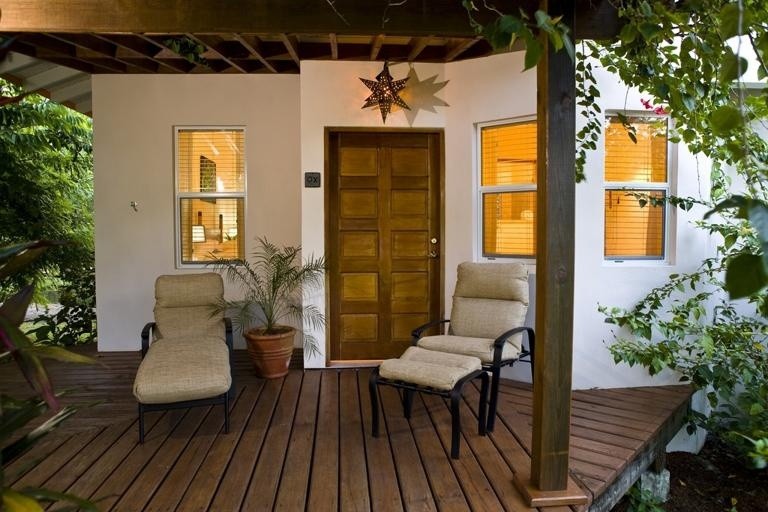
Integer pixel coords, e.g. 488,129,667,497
359,63,414,121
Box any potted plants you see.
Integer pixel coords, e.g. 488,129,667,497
201,239,332,383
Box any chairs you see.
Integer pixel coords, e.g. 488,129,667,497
373,258,535,460
130,275,233,431
